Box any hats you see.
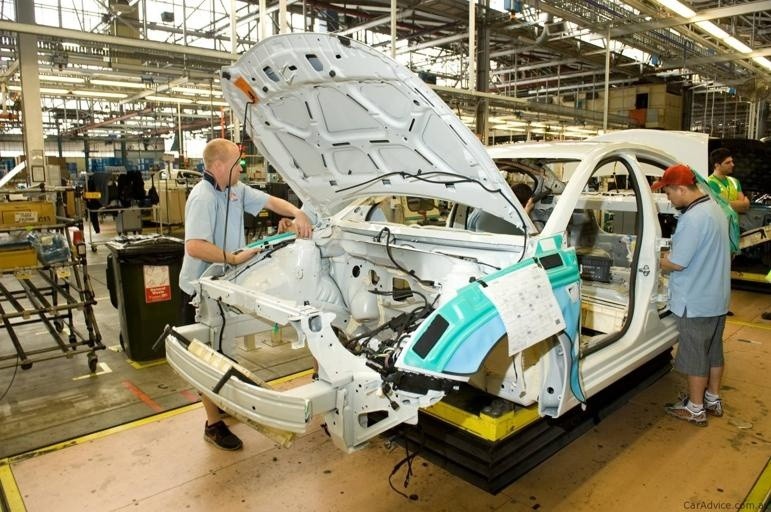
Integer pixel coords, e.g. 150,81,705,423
651,164,696,192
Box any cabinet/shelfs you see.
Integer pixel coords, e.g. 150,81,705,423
0,189,111,374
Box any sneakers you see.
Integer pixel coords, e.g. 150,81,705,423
204,419,243,451
704,396,723,416
664,391,708,427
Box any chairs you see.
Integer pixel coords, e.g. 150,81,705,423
567,205,632,270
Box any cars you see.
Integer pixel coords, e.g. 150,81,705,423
162,31,710,455
140,169,204,200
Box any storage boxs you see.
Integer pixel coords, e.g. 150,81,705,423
0,201,56,227
0,246,38,268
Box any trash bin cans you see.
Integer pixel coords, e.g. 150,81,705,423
103,236,196,361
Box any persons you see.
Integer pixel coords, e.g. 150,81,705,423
650,163,733,428
705,147,751,315
177,137,314,452
276,203,389,234
466,181,536,235
